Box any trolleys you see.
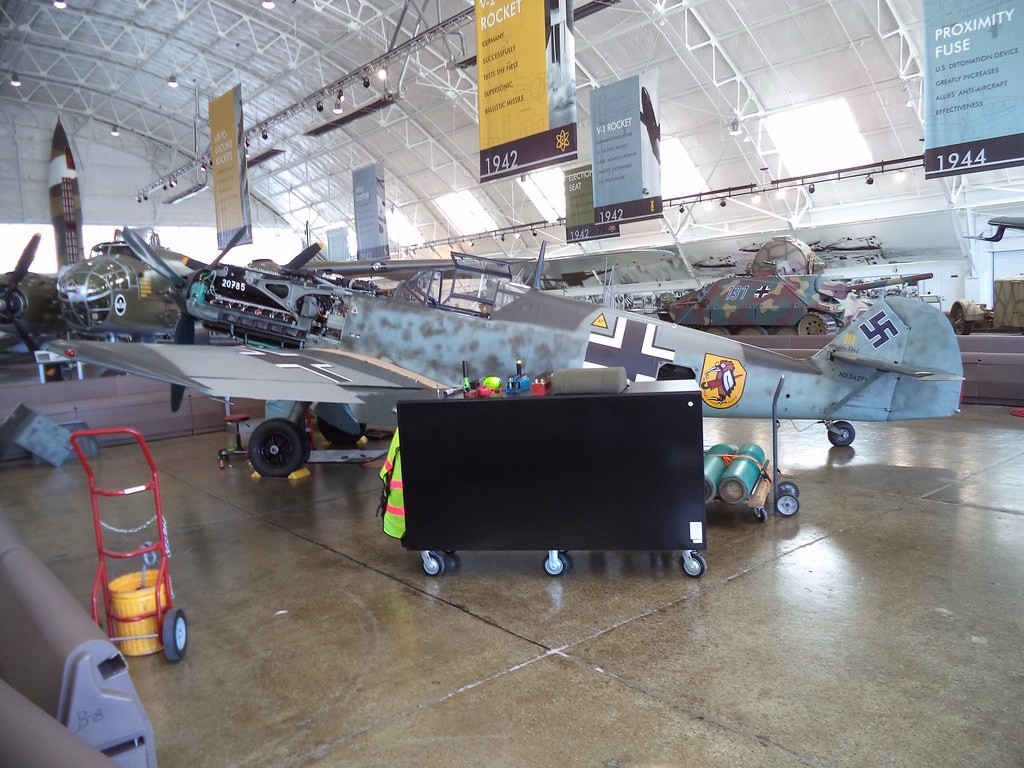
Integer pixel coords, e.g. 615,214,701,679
69,428,185,666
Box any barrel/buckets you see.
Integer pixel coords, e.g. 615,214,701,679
107,569,170,656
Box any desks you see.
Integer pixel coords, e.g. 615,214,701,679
398,378,709,580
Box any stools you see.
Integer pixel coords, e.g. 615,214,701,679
217,414,252,469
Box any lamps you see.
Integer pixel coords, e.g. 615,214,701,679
12,2,878,262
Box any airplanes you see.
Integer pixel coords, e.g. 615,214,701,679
0,229,970,515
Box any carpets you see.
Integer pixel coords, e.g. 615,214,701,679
306,449,389,463
